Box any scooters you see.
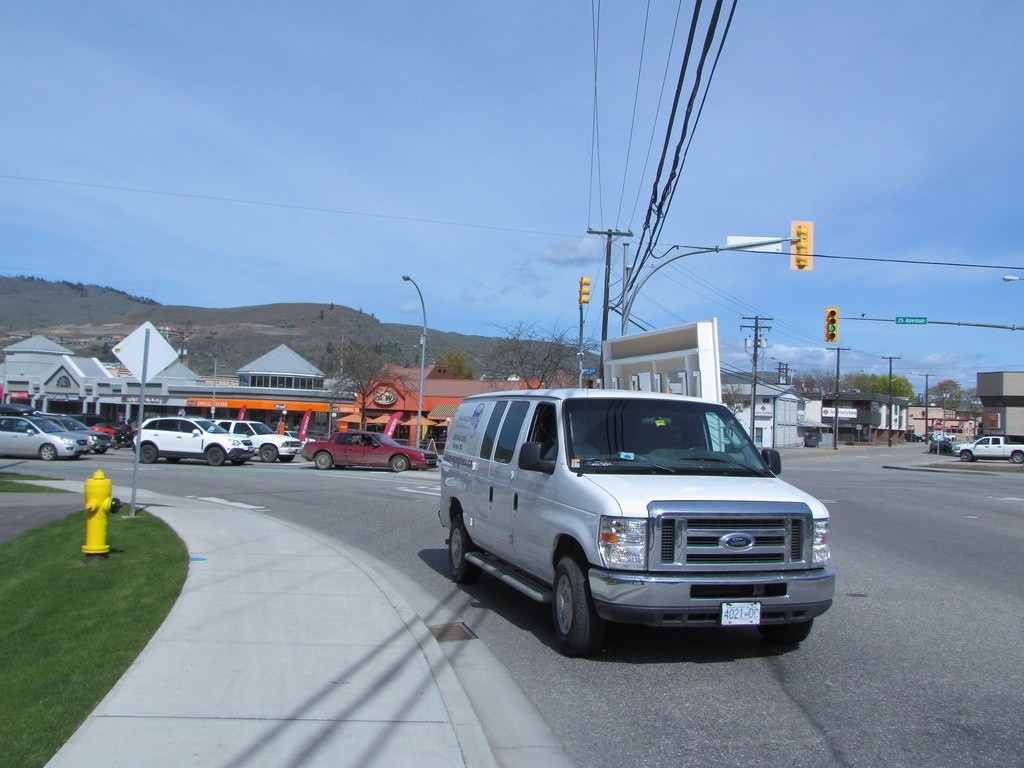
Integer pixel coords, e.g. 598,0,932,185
113,427,133,450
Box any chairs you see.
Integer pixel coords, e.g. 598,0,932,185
543,414,601,461
620,421,682,454
366,436,373,446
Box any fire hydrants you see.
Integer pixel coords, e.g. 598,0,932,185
80,469,123,562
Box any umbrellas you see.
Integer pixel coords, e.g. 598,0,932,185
401,416,438,440
335,412,373,432
435,421,448,426
367,414,403,423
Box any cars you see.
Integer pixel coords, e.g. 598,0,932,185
0,403,91,461
302,431,439,473
929,440,953,453
904,432,926,442
274,430,316,450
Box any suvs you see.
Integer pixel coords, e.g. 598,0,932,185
437,388,837,659
131,415,254,466
31,412,116,454
207,419,302,463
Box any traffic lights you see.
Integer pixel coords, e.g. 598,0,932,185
578,276,591,304
825,306,839,342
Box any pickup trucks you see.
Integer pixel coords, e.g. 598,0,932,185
954,436,1024,464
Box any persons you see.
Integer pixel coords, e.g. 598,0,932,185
544,412,600,459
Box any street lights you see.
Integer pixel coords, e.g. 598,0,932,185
331,412,337,434
402,273,427,449
209,352,217,419
281,410,288,421
